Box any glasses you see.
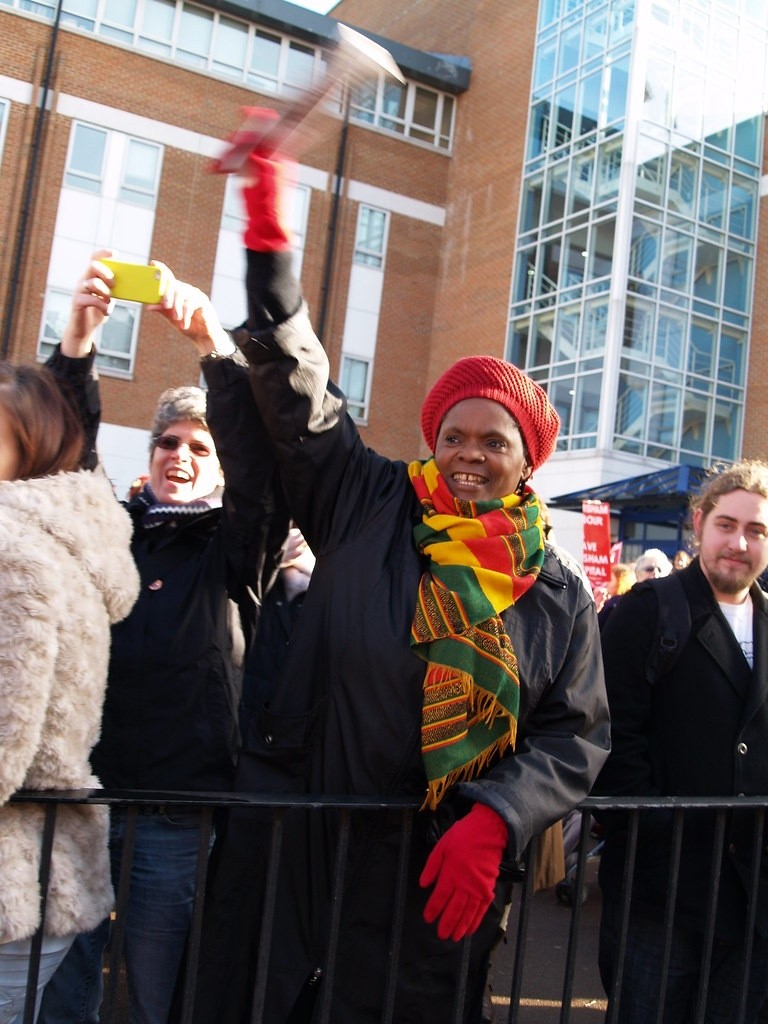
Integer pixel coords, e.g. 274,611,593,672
150,436,212,457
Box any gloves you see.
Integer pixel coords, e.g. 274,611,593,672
203,104,299,252
418,803,510,942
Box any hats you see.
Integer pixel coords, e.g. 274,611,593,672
421,354,561,472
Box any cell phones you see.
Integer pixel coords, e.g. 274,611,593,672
98,258,167,305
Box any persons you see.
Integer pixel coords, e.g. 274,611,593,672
166,107,614,1024
593,467,768,1024
37,249,292,1024
240,518,318,735
0,364,141,1024
549,549,692,906
